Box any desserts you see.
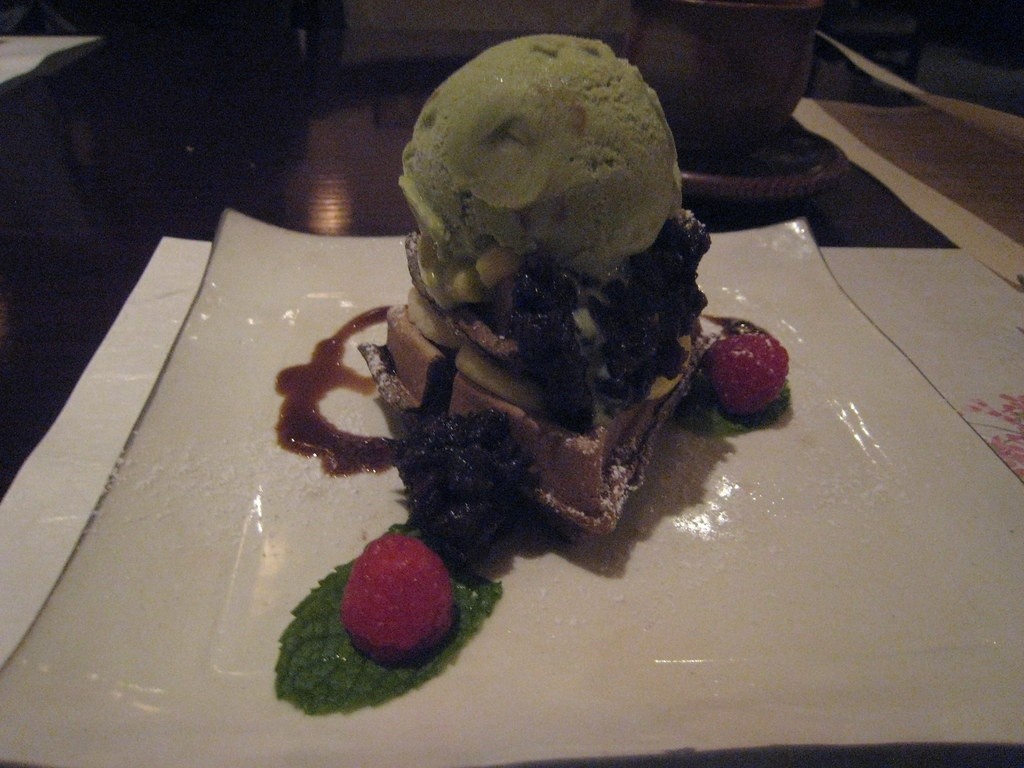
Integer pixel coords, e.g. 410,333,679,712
360,36,715,542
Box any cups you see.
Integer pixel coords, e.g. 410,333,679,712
624,0,825,159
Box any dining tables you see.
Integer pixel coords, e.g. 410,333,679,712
1,99,1024,768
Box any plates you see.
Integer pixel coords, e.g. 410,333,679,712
0,209,1021,768
